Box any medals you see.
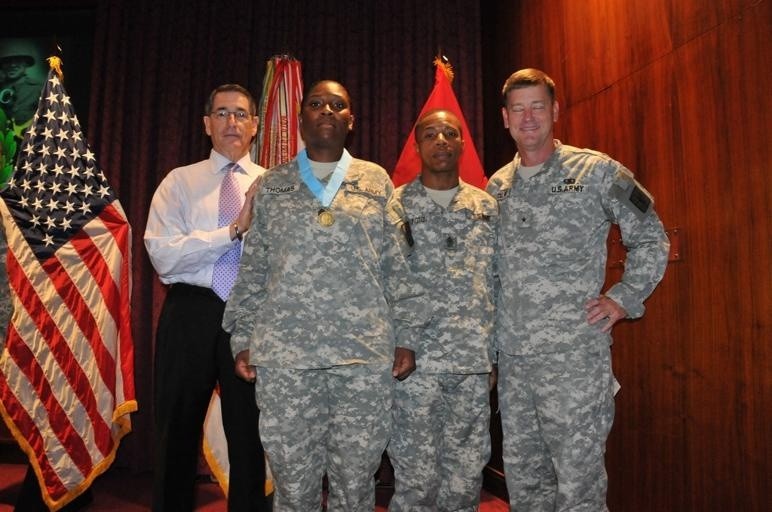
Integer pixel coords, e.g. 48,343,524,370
318,211,335,226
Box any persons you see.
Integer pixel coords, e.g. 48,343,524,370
216,80,433,511
143,81,273,511
482,68,671,511
379,108,499,512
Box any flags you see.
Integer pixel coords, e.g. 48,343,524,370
199,53,304,503
391,53,489,191
0,54,139,510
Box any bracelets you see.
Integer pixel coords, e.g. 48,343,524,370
232,219,244,242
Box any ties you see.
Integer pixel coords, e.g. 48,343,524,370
212,163,243,304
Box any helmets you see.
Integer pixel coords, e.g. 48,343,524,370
0,38,35,70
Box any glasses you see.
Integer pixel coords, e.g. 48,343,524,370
205,108,252,122
